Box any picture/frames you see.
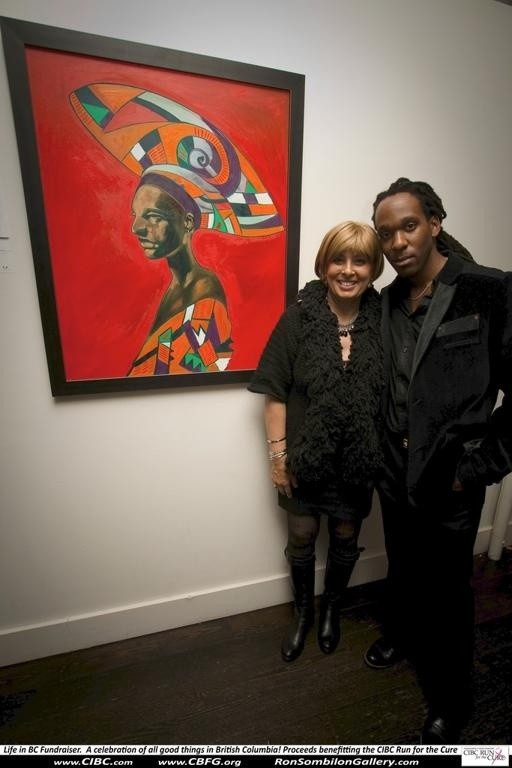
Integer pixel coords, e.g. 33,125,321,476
0,16,305,398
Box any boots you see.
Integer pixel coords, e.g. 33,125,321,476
317,543,363,659
277,544,317,662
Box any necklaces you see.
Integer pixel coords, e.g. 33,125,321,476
338,321,354,337
411,280,432,301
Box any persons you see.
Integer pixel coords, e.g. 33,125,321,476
123,182,239,375
243,218,383,662
359,176,512,744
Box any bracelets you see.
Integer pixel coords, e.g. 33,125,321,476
267,436,287,444
268,448,287,460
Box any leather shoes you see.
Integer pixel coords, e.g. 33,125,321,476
362,629,415,671
419,707,460,743
